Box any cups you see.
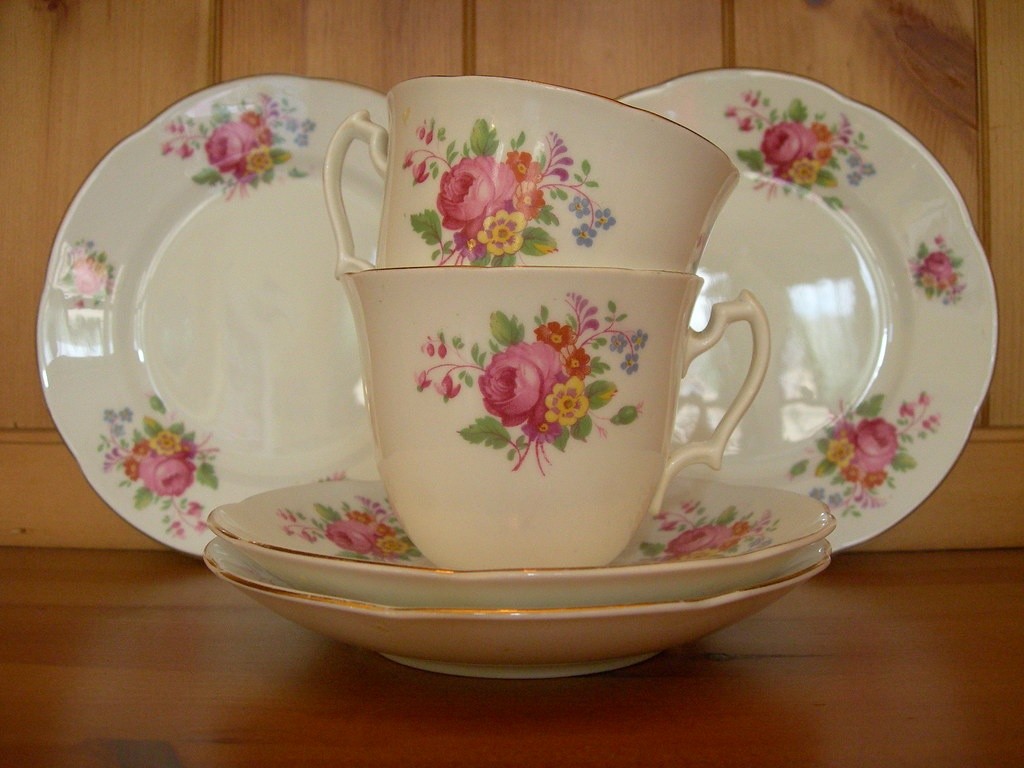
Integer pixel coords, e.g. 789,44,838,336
323,75,740,277
341,266,771,571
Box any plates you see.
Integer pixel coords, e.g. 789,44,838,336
35,75,387,556
613,68,997,553
205,537,833,679
206,479,837,610
